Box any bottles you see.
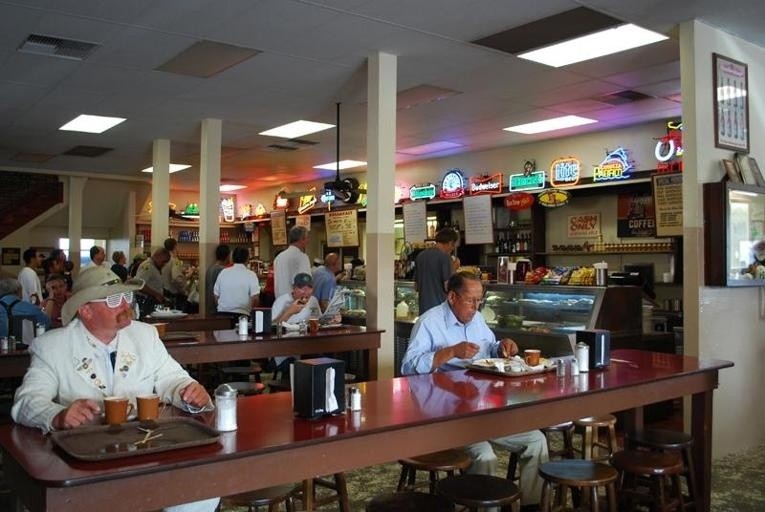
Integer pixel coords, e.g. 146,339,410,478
555,372,589,394
516,217,531,229
213,383,240,431
348,382,367,411
238,315,248,335
570,358,580,376
136,228,173,241
347,410,366,431
556,358,566,377
275,318,282,338
35,322,46,337
496,232,531,253
574,341,590,372
178,231,249,244
299,320,307,335
1,335,16,350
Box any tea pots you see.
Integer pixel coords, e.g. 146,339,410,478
516,256,534,283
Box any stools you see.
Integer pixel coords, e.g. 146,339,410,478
575,415,617,460
652,431,696,498
222,485,297,512
538,460,618,512
506,422,574,483
397,451,472,493
611,450,684,512
222,366,263,394
436,473,521,512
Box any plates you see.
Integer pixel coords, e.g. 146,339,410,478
474,357,513,368
320,323,343,328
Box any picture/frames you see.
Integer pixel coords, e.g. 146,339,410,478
712,52,750,153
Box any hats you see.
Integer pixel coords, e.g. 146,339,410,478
61,266,146,326
295,273,313,287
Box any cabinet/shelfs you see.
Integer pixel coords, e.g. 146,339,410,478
437,202,545,272
135,222,252,260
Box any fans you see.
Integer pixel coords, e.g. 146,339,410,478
280,99,367,207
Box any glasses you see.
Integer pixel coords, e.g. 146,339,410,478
89,292,134,307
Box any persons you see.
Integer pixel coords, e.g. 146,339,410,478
400,271,550,512
131,227,353,379
9,266,221,512
0,245,148,338
414,228,460,316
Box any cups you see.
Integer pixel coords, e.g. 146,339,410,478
103,395,134,424
135,393,167,421
154,322,168,337
523,348,542,366
592,263,609,286
309,319,319,334
